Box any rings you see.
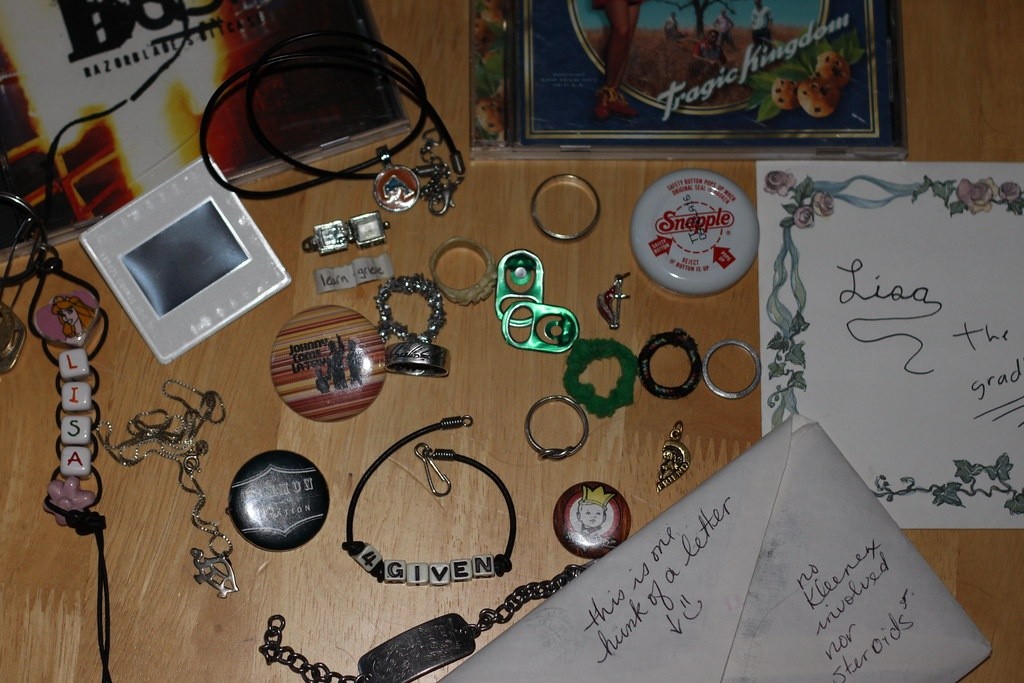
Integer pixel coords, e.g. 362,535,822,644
639,330,702,399
566,339,639,416
429,236,496,305
374,277,446,344
529,173,601,242
702,340,762,399
524,395,589,459
384,341,448,376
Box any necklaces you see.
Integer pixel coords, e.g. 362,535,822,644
0,192,112,683
96,378,240,597
197,28,465,217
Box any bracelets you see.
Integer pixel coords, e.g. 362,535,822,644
342,414,518,586
258,556,595,682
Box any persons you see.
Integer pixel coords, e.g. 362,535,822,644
590,0,645,118
664,1,773,107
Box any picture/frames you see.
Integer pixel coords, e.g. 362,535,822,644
465,0,908,165
77,156,291,365
